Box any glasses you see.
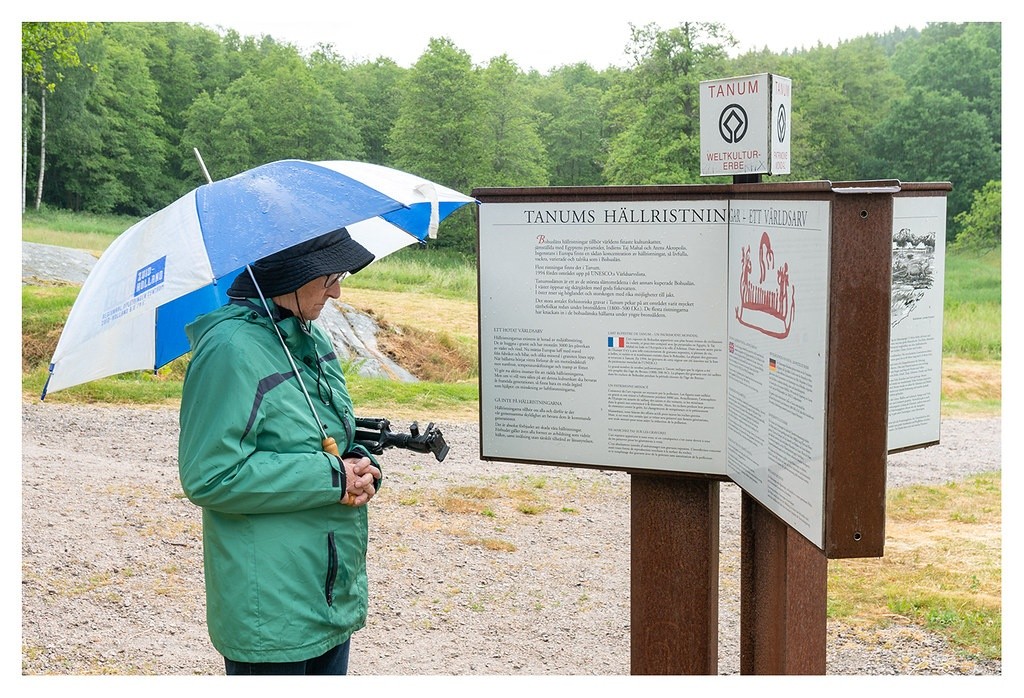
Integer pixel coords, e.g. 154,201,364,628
324,271,347,289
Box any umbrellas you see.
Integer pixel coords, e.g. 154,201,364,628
41,146,482,507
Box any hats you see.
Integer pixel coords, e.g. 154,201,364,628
226,227,375,299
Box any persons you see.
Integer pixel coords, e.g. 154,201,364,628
179,225,383,676
